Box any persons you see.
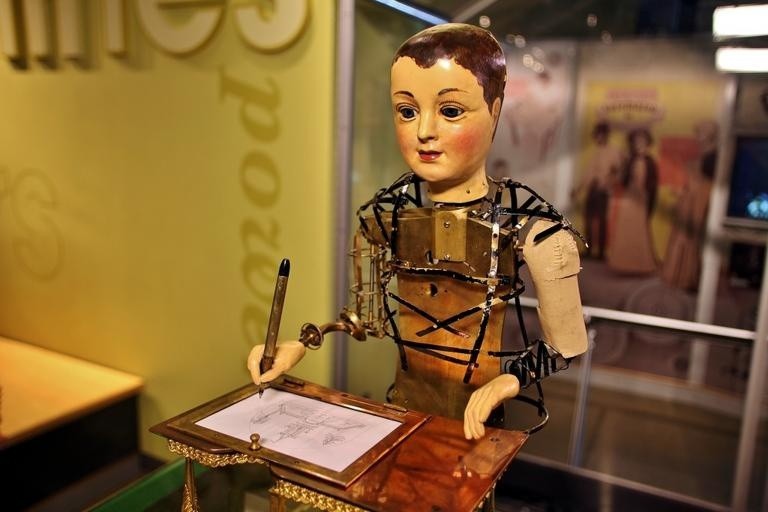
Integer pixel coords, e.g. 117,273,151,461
532,71,564,177
244,20,592,445
606,125,663,278
577,120,625,263
672,118,719,299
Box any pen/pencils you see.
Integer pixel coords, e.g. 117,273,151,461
254,261,290,399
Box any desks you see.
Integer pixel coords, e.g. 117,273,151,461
0,334,145,512
